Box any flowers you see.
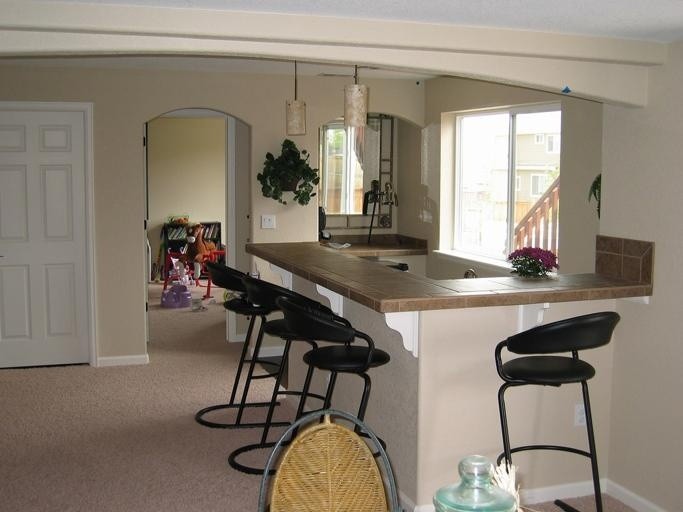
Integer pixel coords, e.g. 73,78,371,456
507,247,560,280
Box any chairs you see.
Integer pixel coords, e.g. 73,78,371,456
228,275,354,476
495,311,621,512
196,259,293,428
164,252,199,290
257,410,400,512
275,293,390,444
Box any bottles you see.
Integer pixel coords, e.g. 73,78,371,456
429,454,518,512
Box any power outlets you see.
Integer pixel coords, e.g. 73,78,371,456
261,214,276,229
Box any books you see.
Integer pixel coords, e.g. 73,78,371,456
168,223,221,269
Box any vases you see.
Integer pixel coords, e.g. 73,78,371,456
525,264,539,280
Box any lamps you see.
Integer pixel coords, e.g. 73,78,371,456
286,59,306,135
344,64,368,126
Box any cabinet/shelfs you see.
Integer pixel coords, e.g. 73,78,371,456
164,222,221,278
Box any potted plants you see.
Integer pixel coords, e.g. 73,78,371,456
256,139,321,205
